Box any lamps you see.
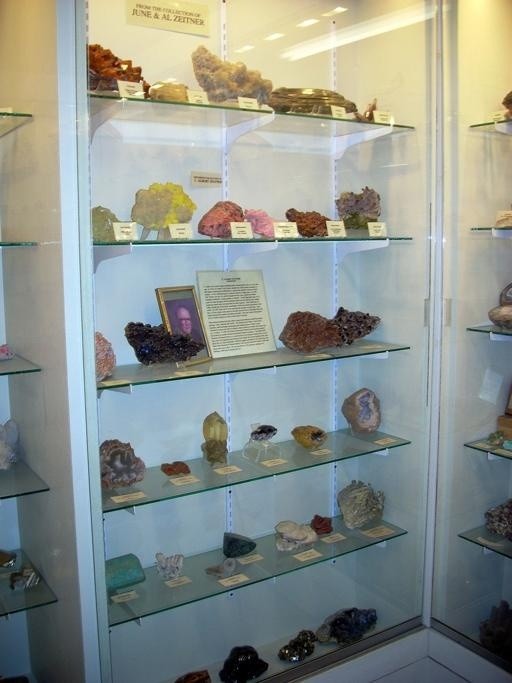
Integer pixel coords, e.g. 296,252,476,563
280,3,431,67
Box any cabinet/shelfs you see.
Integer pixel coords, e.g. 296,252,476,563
76,0,443,683
2,3,100,683
427,0,510,683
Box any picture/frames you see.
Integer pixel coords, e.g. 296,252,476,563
152,284,209,369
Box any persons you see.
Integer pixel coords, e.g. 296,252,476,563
173,307,203,345
